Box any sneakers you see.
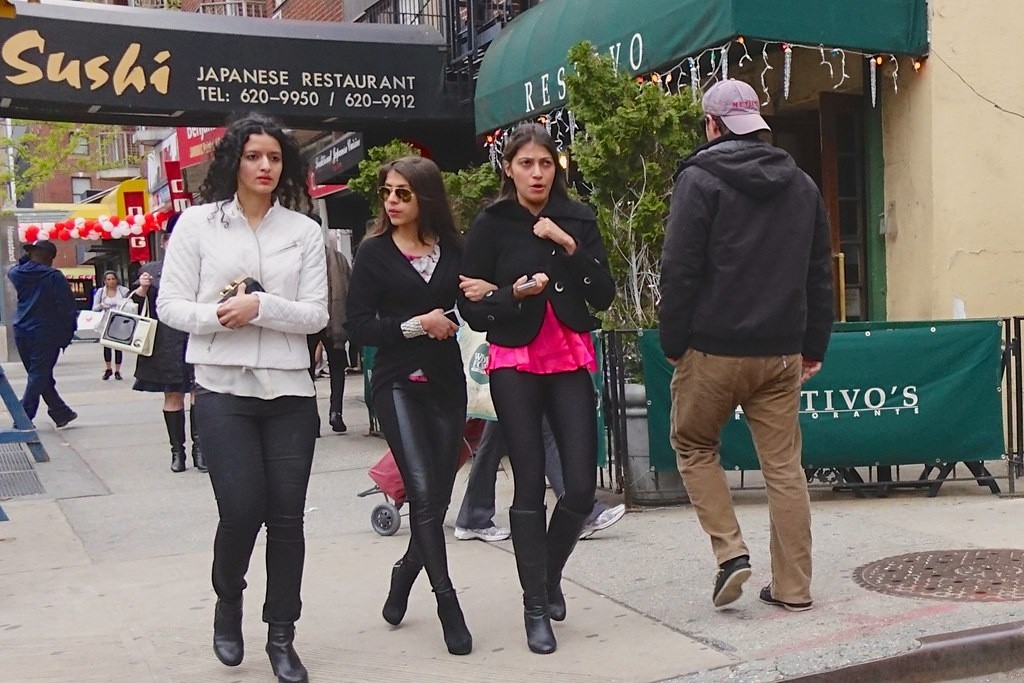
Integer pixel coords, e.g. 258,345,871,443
578,502,627,539
454,525,511,542
759,580,813,612
115,371,122,380
102,369,113,380
713,555,751,607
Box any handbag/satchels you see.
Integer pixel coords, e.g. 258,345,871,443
455,323,500,420
74,309,105,339
100,288,157,356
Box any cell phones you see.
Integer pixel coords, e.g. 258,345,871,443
515,280,536,291
427,309,465,338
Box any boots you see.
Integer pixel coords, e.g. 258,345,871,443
508,504,557,653
190,405,209,472
547,490,600,621
265,621,308,683
213,579,248,666
163,408,186,472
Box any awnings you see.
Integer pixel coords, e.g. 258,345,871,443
475,1,928,135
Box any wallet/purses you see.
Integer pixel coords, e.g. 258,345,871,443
217,277,266,304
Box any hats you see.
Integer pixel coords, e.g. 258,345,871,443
162,214,180,234
23,240,57,258
702,78,772,136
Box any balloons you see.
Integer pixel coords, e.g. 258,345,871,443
17,211,174,242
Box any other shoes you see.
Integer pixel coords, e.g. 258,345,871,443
57,412,78,426
330,412,347,431
353,367,360,374
319,369,330,378
13,423,36,430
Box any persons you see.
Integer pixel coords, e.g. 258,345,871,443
157,117,331,683
458,124,617,654
92,271,130,380
660,77,836,612
7,240,78,428
131,211,207,472
307,211,353,439
453,413,627,541
349,157,499,656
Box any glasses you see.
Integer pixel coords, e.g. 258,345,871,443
377,185,418,202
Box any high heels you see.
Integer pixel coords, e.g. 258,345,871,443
434,589,473,655
382,552,422,626
